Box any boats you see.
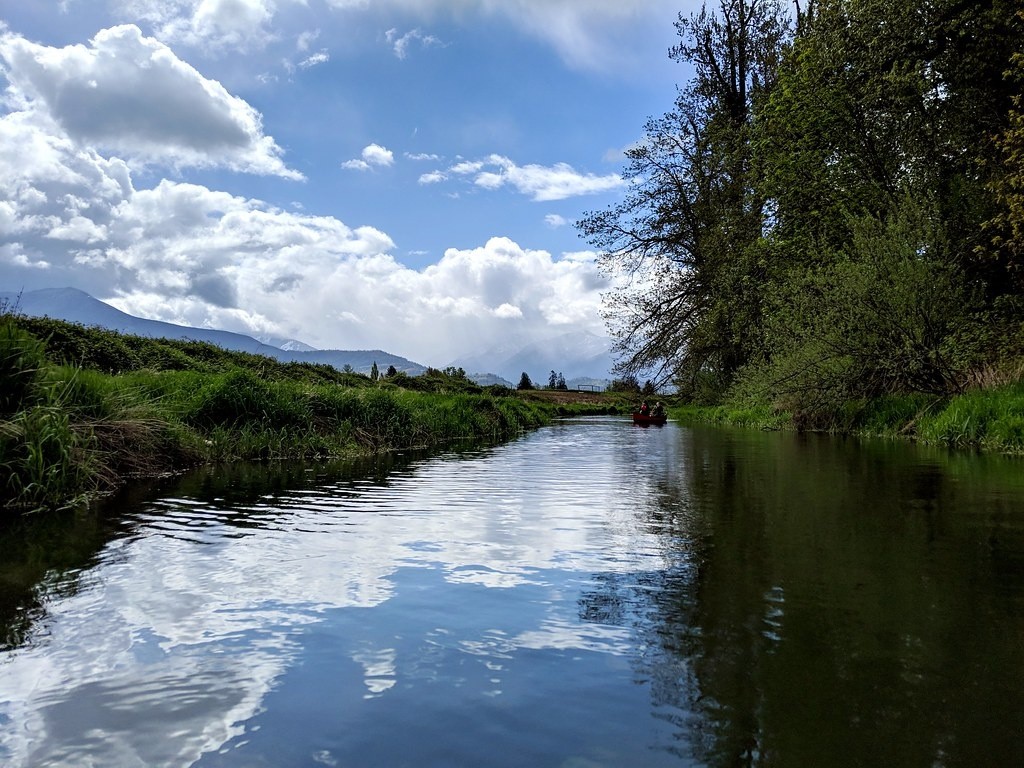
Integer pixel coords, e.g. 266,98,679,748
652,413,666,425
634,413,648,423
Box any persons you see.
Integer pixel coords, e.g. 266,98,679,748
651,401,663,416
638,400,650,415
